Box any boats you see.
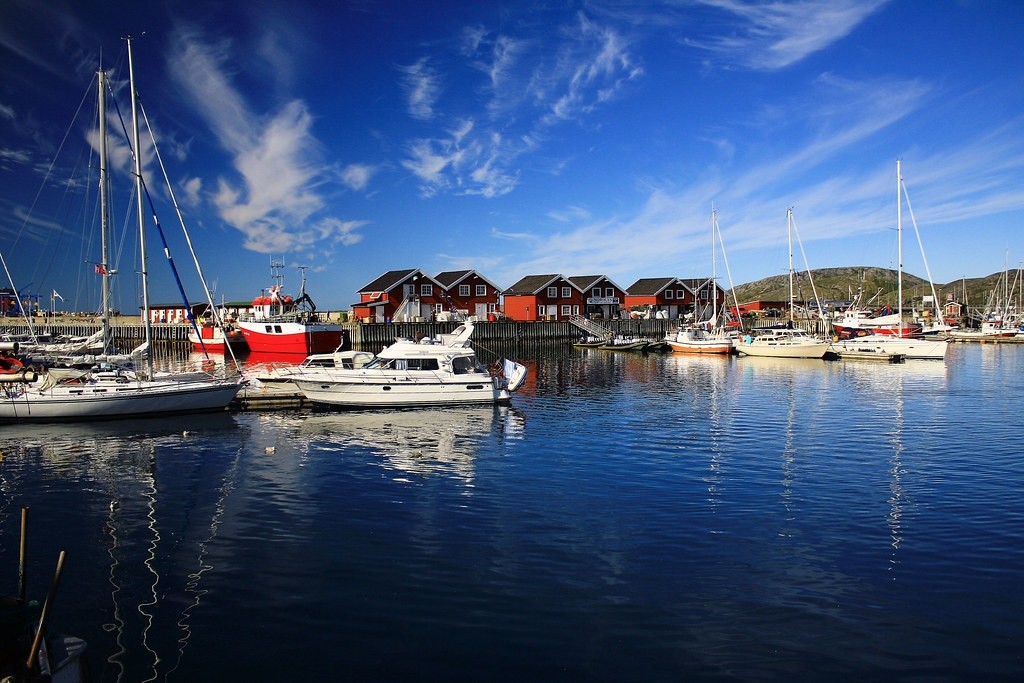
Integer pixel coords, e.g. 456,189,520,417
254,331,375,393
278,311,528,408
186,278,248,354
572,338,666,351
830,270,923,339
236,252,343,354
663,286,733,353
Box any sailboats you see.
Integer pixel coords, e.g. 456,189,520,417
922,247,1024,338
827,157,950,359
0,32,252,423
736,207,832,357
709,201,750,347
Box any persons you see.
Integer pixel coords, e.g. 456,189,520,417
309,314,317,323
380,360,386,369
